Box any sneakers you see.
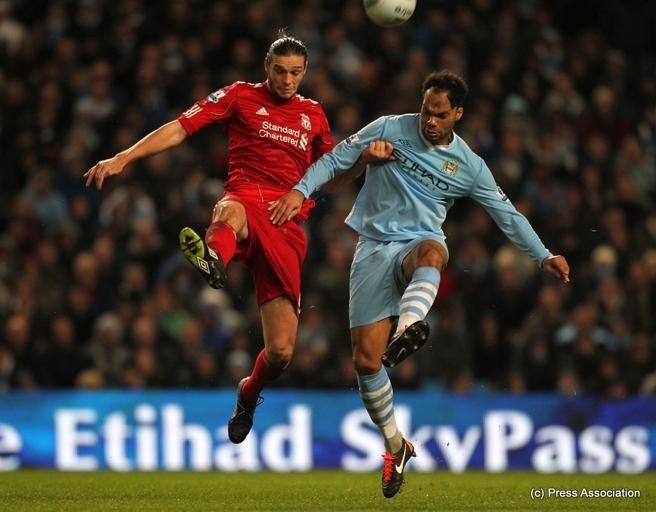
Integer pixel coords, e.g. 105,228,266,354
176,225,229,290
228,376,265,445
380,320,431,368
380,437,417,498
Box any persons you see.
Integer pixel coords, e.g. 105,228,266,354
268,68,571,501
1,1,656,398
83,27,397,444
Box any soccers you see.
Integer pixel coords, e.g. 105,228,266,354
361,0,417,31
